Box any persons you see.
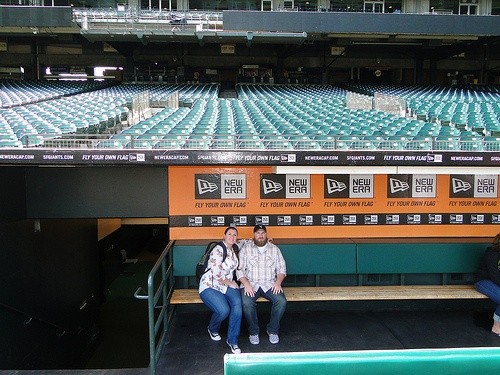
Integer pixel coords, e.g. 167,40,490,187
113,239,151,282
198,227,273,354
473,233,500,337
236,224,287,345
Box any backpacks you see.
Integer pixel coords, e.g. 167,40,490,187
195,241,239,286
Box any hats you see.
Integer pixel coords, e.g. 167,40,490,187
254,224,266,233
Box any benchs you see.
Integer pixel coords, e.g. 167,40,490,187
168,242,493,304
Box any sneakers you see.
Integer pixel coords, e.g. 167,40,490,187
226,340,242,354
267,330,280,344
207,328,221,341
249,334,260,345
